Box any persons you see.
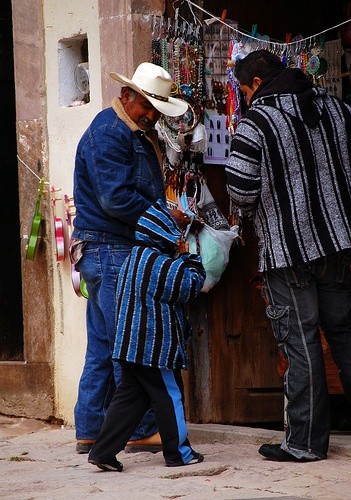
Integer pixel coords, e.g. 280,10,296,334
225,49,351,462
87,198,208,471
70,62,188,455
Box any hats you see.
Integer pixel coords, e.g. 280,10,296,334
109,62,190,118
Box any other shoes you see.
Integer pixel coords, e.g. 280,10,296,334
124,432,164,453
258,442,307,463
76,439,97,454
187,452,204,463
87,456,123,472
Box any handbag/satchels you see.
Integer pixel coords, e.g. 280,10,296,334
256,281,347,396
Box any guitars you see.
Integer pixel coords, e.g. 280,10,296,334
26,177,82,298
